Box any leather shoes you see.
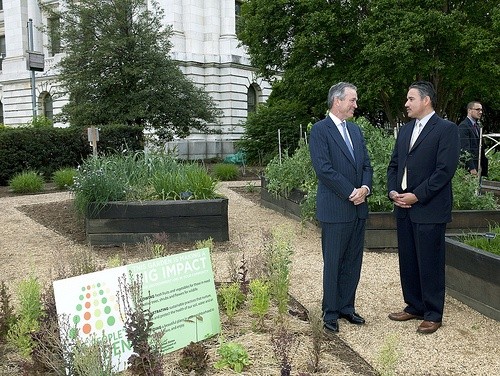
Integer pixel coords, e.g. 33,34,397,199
417,320,441,333
324,320,339,332
339,312,365,324
388,311,425,321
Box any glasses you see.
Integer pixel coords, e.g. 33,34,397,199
470,107,483,111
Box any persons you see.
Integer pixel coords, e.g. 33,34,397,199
461,101,489,180
386,81,460,333
309,82,375,330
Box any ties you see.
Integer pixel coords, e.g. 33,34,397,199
474,123,479,138
401,122,421,191
341,122,355,164
